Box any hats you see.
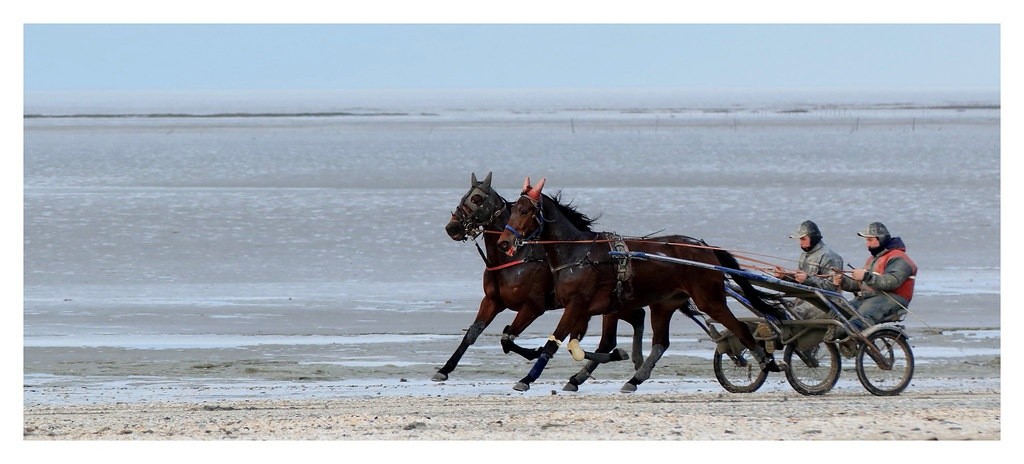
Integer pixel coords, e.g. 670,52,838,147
857,222,891,244
789,220,821,238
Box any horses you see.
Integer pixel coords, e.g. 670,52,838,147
431,171,790,393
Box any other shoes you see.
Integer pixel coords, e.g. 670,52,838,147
824,310,842,340
758,323,776,354
709,325,729,354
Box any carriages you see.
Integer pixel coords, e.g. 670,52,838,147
432,170,917,397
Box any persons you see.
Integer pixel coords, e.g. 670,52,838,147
779,221,918,359
708,220,844,355
